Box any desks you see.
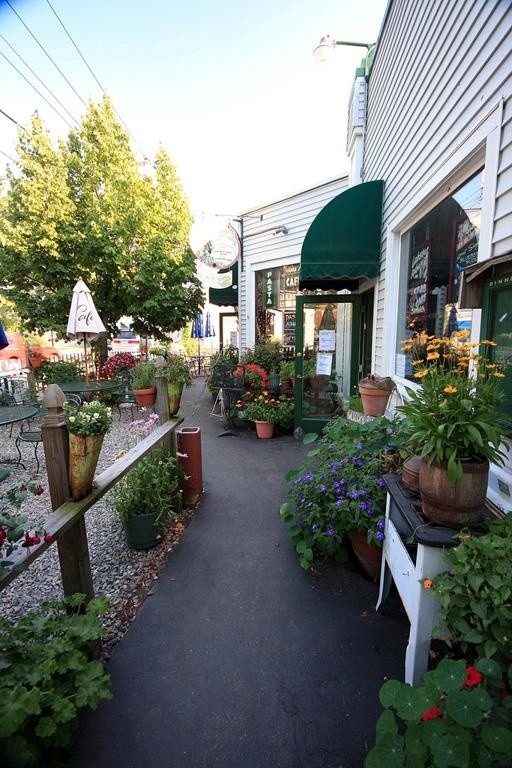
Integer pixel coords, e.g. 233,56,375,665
375,474,500,691
0,403,37,471
57,379,122,422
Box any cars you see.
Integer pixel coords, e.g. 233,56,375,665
111,328,154,355
0,330,62,368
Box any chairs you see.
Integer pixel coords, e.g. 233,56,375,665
4,386,81,473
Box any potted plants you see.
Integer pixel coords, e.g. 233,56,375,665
129,356,193,415
107,447,181,551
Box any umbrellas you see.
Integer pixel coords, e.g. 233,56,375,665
443,306,459,337
190,305,215,375
0,322,9,351
66,276,107,383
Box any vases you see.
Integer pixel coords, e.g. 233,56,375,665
282,375,328,391
401,452,489,529
255,421,274,439
66,433,104,502
359,379,392,418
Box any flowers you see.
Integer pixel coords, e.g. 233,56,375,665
63,400,113,438
398,329,512,486
237,390,295,429
